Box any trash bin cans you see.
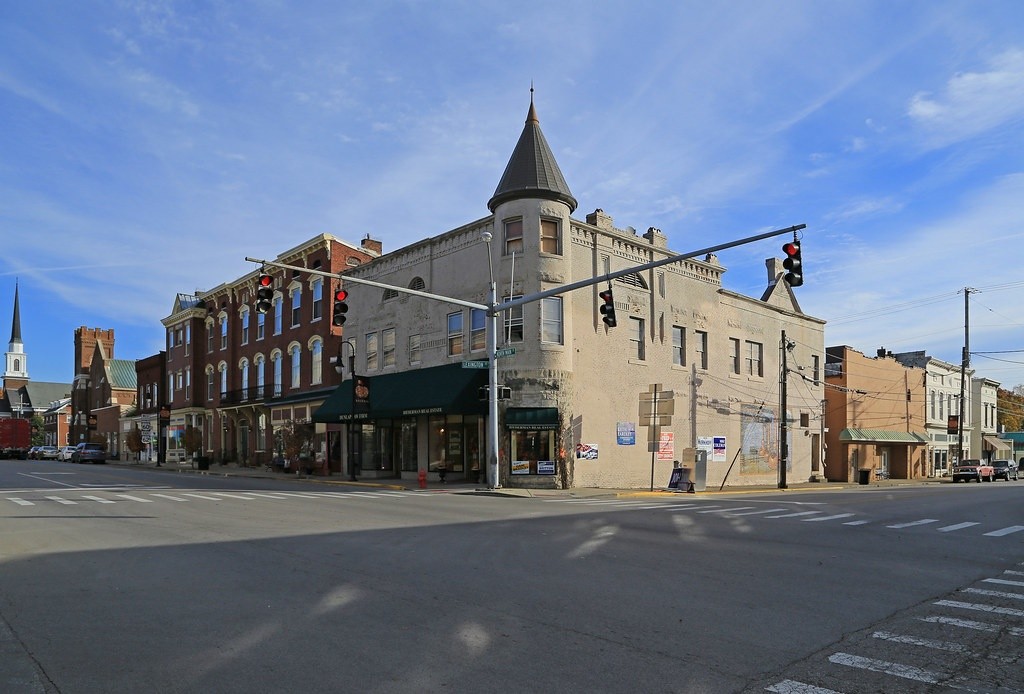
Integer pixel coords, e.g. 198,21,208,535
682,447,708,492
858,469,872,485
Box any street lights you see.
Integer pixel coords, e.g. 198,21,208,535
479,231,501,489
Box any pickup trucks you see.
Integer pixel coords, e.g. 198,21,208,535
952,459,996,484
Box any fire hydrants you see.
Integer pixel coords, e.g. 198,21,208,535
417,468,427,488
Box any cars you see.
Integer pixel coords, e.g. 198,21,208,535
57,445,76,462
28,447,41,460
70,442,106,465
38,446,59,459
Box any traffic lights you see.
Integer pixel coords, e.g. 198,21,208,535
599,289,616,327
255,272,274,313
333,287,349,327
782,239,803,287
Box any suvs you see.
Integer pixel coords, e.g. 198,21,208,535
989,460,1020,481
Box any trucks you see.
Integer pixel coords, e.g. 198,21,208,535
0,418,31,461
